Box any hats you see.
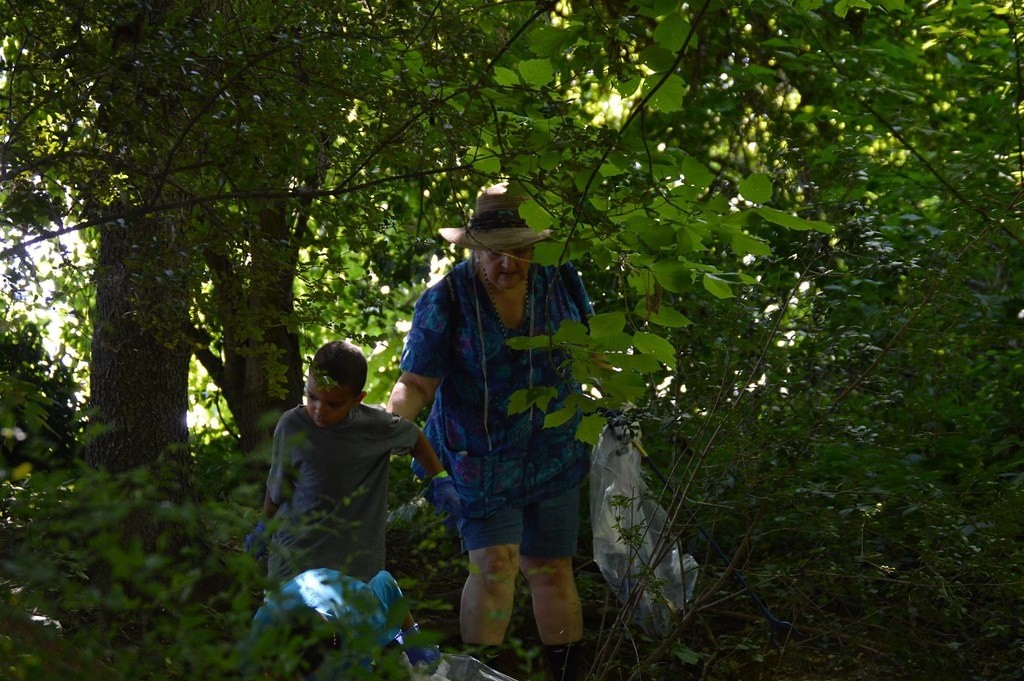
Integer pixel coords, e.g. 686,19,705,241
438,182,554,250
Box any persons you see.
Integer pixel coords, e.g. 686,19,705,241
241,569,438,681
385,191,621,681
245,340,462,601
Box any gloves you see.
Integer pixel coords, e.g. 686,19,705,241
400,623,442,675
425,477,461,534
245,521,271,561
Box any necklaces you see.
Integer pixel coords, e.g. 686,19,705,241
483,263,530,359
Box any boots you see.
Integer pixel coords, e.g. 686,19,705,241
463,642,501,668
545,640,582,680
